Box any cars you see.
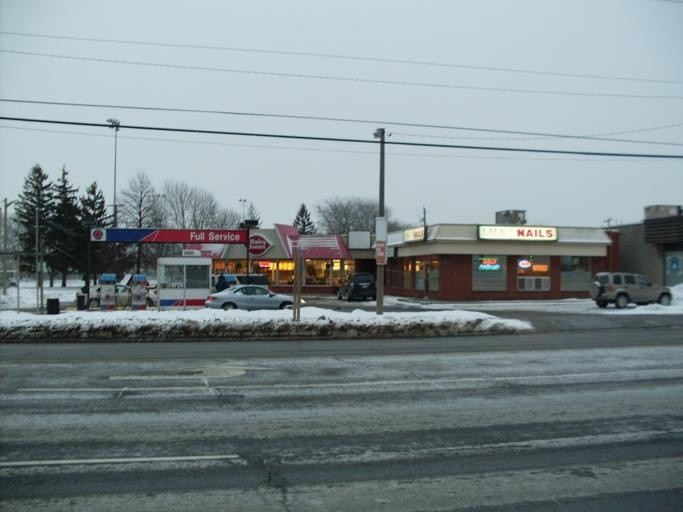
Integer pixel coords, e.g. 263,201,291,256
74,279,131,309
202,283,308,311
335,270,376,302
145,284,157,307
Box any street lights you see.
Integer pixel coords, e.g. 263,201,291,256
105,115,122,233
1,197,22,297
238,197,248,226
369,125,386,315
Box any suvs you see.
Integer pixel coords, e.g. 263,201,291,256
589,271,673,309
209,272,271,294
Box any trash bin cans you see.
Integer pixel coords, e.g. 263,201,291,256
46,298,60,315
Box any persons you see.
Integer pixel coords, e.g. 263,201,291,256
216,274,230,292
